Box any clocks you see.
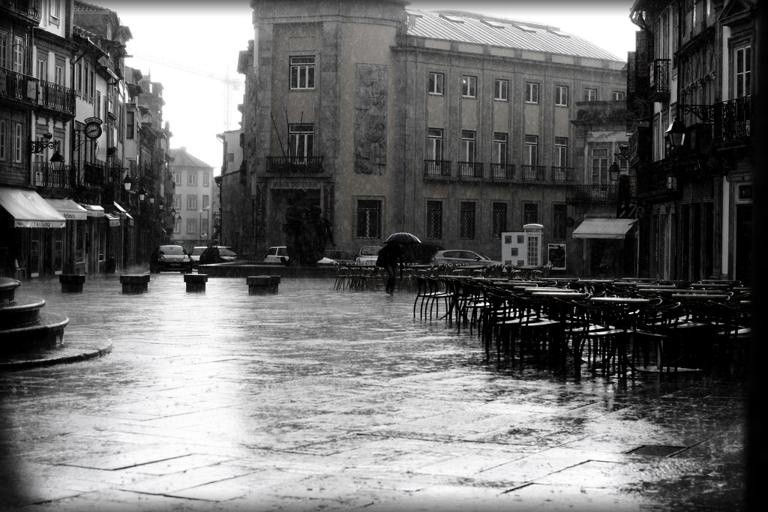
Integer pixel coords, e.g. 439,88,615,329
84,122,101,138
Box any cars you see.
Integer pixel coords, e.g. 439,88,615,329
354,245,383,267
263,246,339,266
149,243,193,274
432,249,494,266
189,245,244,270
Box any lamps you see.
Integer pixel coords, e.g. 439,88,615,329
662,113,691,160
607,153,633,171
30,139,66,163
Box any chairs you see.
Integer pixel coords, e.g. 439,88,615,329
414,274,753,391
334,264,559,296
